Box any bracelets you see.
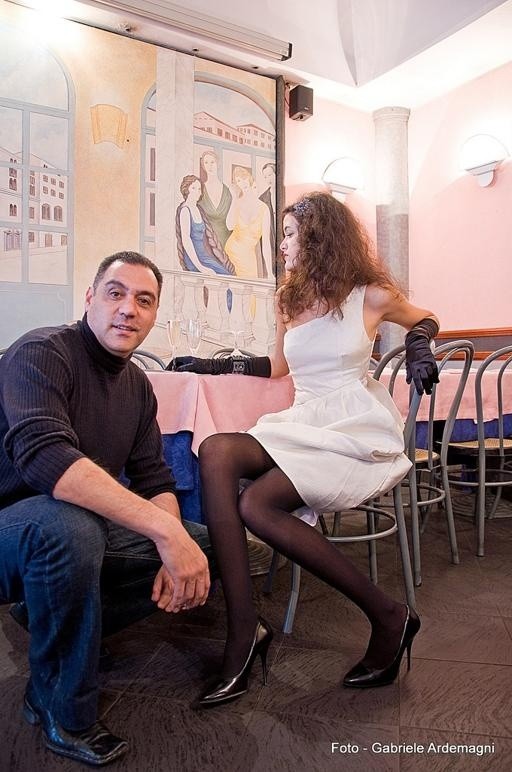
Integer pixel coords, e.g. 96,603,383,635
227,354,246,376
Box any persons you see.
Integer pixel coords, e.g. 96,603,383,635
224,166,276,318
174,174,236,313
258,163,280,278
0,251,223,764
161,189,441,707
199,150,234,252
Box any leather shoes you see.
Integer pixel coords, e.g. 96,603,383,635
23,682,130,765
8,598,113,675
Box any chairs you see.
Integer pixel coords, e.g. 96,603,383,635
0,340,512,634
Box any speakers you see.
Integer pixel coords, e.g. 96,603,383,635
289,85,313,122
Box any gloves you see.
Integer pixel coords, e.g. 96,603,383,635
405,318,440,395
164,355,271,378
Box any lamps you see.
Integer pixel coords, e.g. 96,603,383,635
464,158,504,187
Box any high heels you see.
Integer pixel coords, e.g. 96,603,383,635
189,616,273,710
343,603,421,688
